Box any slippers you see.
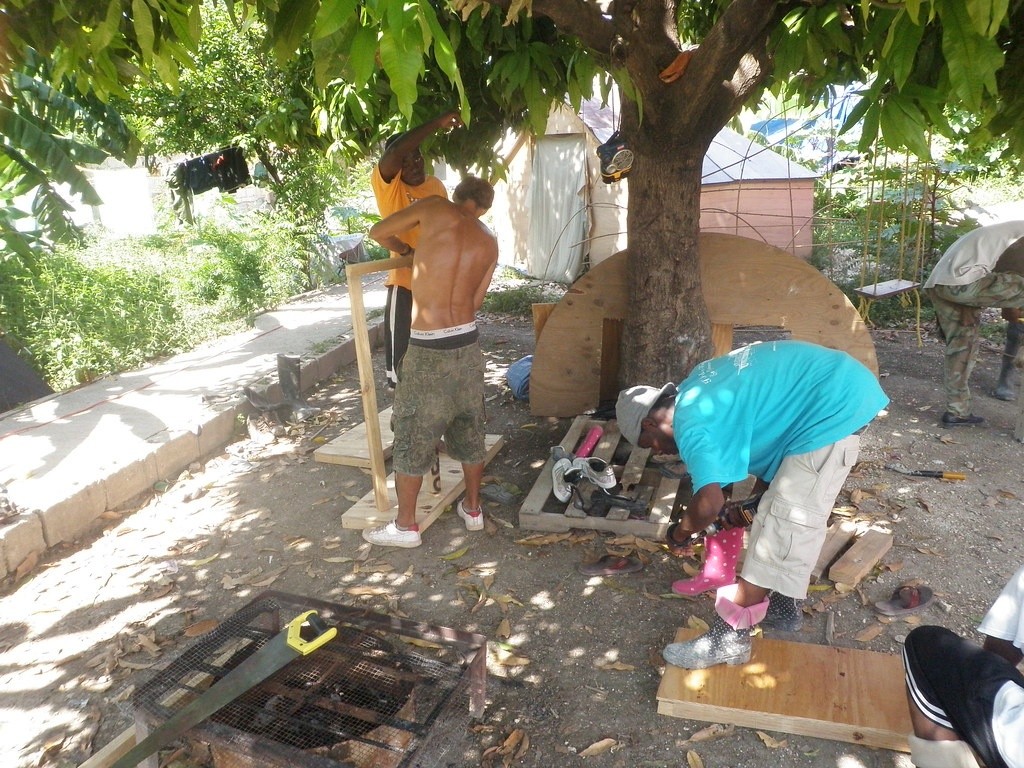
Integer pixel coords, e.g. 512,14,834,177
875,583,933,616
577,555,643,576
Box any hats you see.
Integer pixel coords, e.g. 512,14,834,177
615,382,676,448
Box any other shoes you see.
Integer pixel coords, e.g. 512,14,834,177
645,448,691,479
246,412,286,445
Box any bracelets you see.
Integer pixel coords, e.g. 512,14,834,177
666,523,692,547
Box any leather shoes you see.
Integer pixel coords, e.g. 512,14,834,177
942,412,984,426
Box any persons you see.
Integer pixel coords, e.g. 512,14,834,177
993,308,1024,403
370,113,463,450
616,341,890,670
361,176,499,550
903,565,1023,767
922,221,1023,428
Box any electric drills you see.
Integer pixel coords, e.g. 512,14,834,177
690,492,764,545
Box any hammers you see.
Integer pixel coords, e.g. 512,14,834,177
553,424,604,462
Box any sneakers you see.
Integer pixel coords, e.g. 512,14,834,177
457,497,484,531
552,458,582,503
573,457,616,489
362,516,422,548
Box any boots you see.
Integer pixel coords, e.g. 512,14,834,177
276,354,322,415
662,585,769,670
759,591,805,632
673,525,743,597
994,352,1017,401
244,387,311,422
